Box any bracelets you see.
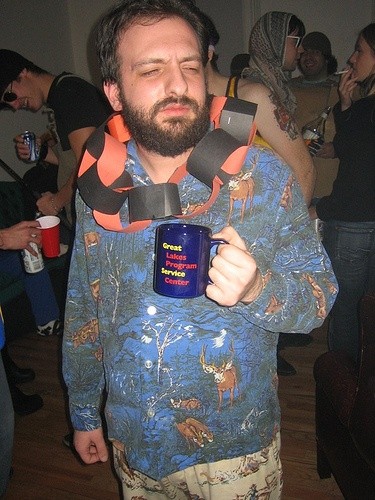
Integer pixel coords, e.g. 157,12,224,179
51,192,63,213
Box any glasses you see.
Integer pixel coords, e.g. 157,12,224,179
287,36,303,49
0,81,17,111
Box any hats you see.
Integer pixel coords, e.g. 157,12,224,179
0,49,29,97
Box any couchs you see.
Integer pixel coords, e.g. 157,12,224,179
0,181,72,342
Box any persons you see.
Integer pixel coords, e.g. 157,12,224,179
0,221,42,500
198,11,375,376
60,0,339,500
0,48,112,448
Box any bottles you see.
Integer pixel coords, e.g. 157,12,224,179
301,105,333,156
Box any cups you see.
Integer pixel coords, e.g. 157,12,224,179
153,223,229,299
35,215,61,257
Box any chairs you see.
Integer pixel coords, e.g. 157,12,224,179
313,293,375,500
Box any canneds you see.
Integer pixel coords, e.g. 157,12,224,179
21,130,40,162
21,234,44,273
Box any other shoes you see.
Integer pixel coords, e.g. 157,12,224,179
276,354,296,377
37,318,64,336
277,332,314,350
4,372,44,416
0,346,35,384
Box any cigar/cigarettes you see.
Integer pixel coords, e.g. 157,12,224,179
335,70,348,75
